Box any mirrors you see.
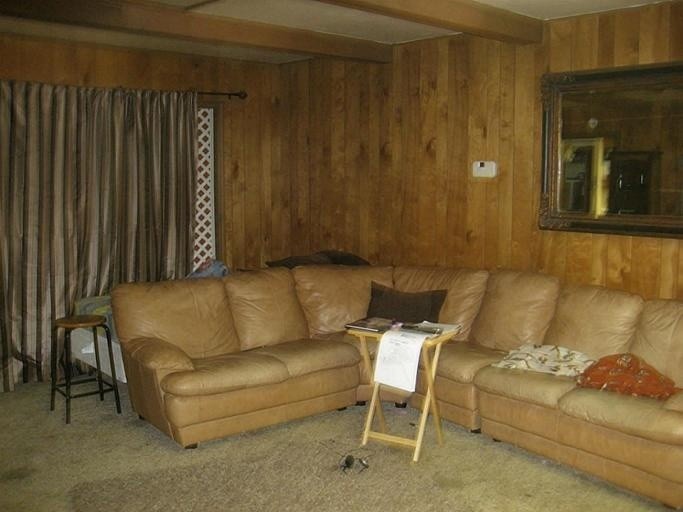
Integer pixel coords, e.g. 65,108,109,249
537,60,683,240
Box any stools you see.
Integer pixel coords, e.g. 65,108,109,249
50,314,121,425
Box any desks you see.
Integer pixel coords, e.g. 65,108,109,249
347,324,462,462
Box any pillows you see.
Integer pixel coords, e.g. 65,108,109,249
265,250,371,269
368,281,449,324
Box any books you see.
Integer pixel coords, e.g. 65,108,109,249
344,317,392,332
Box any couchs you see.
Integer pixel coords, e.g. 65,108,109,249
110,265,682,511
